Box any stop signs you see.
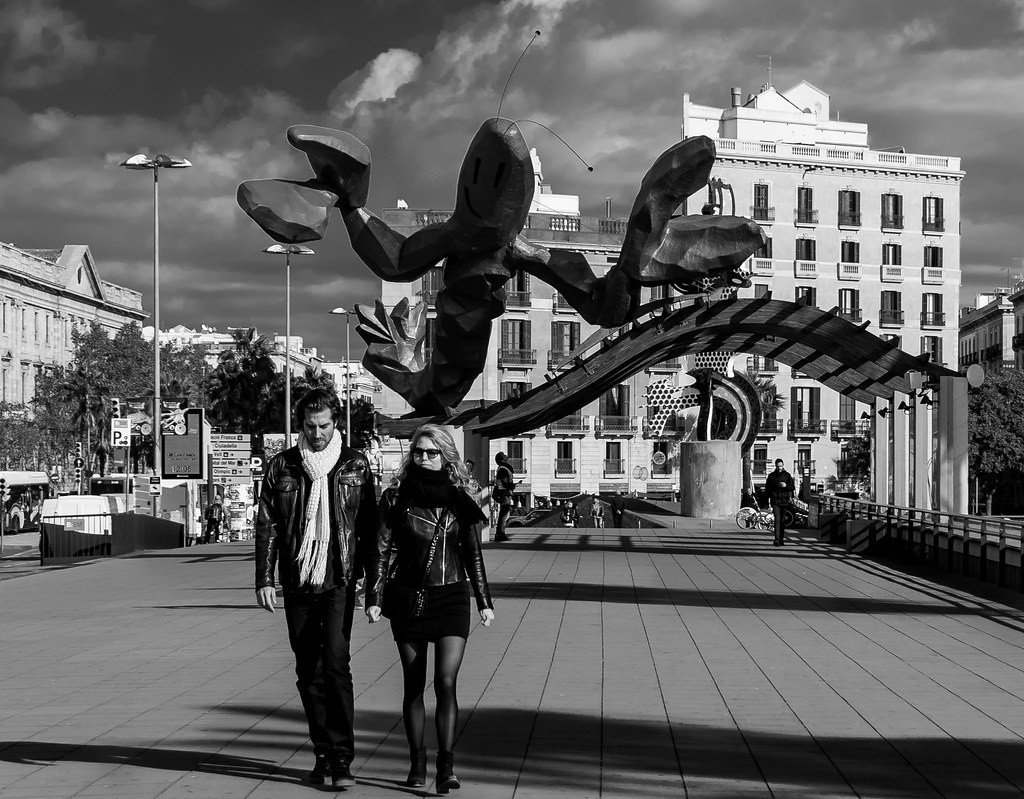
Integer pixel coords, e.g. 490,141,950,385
50,474,59,484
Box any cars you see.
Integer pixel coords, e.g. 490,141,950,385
507,510,553,528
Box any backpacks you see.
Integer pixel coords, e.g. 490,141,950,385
210,502,223,523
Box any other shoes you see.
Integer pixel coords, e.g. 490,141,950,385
494,536,512,543
206,542,208,544
216,540,221,542
330,760,356,787
304,755,331,785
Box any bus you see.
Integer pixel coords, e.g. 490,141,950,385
87,473,154,495
0,471,61,533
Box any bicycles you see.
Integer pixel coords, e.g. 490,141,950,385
736,491,795,530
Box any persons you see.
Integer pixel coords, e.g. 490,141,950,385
254,390,377,788
611,491,625,528
495,452,516,542
364,422,496,793
560,500,583,528
589,496,605,528
739,486,758,529
204,494,228,545
764,458,795,546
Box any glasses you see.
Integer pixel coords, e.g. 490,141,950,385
412,447,441,459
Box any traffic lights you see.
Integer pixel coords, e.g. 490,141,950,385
75,469,80,484
111,398,121,419
0,478,5,495
74,442,81,457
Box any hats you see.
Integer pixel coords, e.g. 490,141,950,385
216,495,221,500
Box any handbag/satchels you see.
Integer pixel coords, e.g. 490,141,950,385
380,579,427,620
492,487,505,503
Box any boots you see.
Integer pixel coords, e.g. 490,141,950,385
435,751,460,794
407,746,428,787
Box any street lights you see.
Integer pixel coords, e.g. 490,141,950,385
118,153,192,476
329,304,366,447
264,242,315,449
